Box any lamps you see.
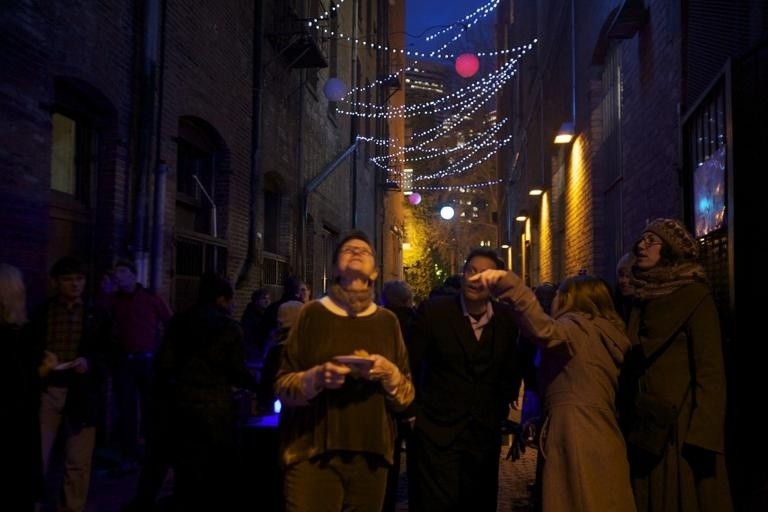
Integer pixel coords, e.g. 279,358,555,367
554,122,574,145
454,25,479,78
322,77,345,102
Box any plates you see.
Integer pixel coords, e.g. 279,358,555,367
53,360,80,372
331,355,375,369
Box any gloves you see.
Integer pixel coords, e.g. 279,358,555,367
505,432,525,462
682,443,717,479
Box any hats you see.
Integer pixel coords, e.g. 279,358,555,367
616,251,637,275
277,300,304,328
643,217,700,261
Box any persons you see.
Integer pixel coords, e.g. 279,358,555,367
0,248,767,511
611,213,733,511
271,230,416,511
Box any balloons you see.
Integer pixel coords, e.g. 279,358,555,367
453,52,482,79
323,78,349,103
